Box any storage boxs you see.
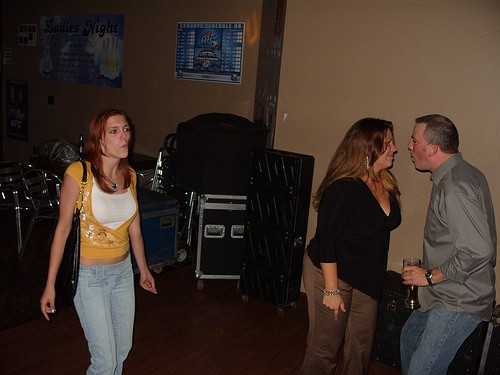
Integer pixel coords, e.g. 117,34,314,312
374,270,500,375
131,187,179,275
196,192,246,280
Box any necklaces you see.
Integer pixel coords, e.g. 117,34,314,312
112,183,117,188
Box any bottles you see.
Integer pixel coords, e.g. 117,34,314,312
78,134,84,155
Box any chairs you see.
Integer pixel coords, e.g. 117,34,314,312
0,161,63,258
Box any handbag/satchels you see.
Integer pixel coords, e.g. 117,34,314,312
55,212,82,302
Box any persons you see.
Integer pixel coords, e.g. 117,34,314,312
298,117,402,375
40,107,158,375
400,114,497,375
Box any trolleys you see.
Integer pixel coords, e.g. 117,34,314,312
132,132,199,275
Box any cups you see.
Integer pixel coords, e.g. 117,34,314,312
401,258,421,310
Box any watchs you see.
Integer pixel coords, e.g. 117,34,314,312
425,269,436,286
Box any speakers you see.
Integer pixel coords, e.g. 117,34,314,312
372,271,489,375
239,147,314,307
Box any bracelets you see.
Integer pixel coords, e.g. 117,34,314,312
323,289,340,295
